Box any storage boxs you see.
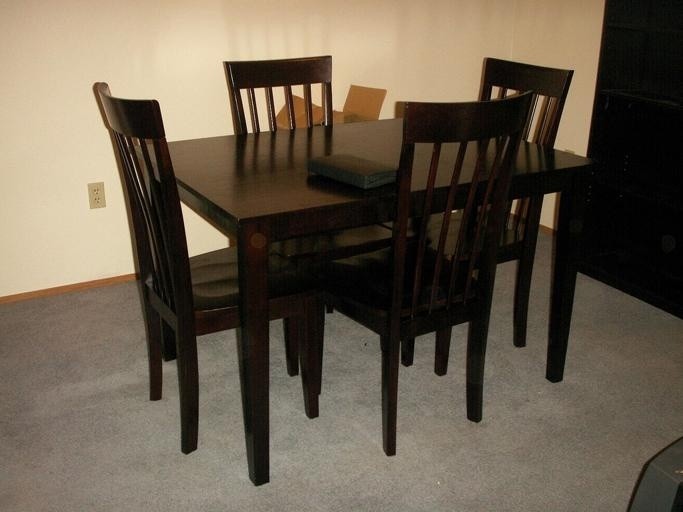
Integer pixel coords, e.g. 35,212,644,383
275,84,387,133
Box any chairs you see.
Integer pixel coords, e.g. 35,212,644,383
221,55,392,376
304,89,534,454
400,57,572,366
91,82,322,457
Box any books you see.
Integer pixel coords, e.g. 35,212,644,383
306,152,399,190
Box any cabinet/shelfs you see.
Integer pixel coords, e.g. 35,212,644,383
571,0,682,322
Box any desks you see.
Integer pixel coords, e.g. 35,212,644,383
130,117,598,487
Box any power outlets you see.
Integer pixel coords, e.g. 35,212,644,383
86,180,106,211
